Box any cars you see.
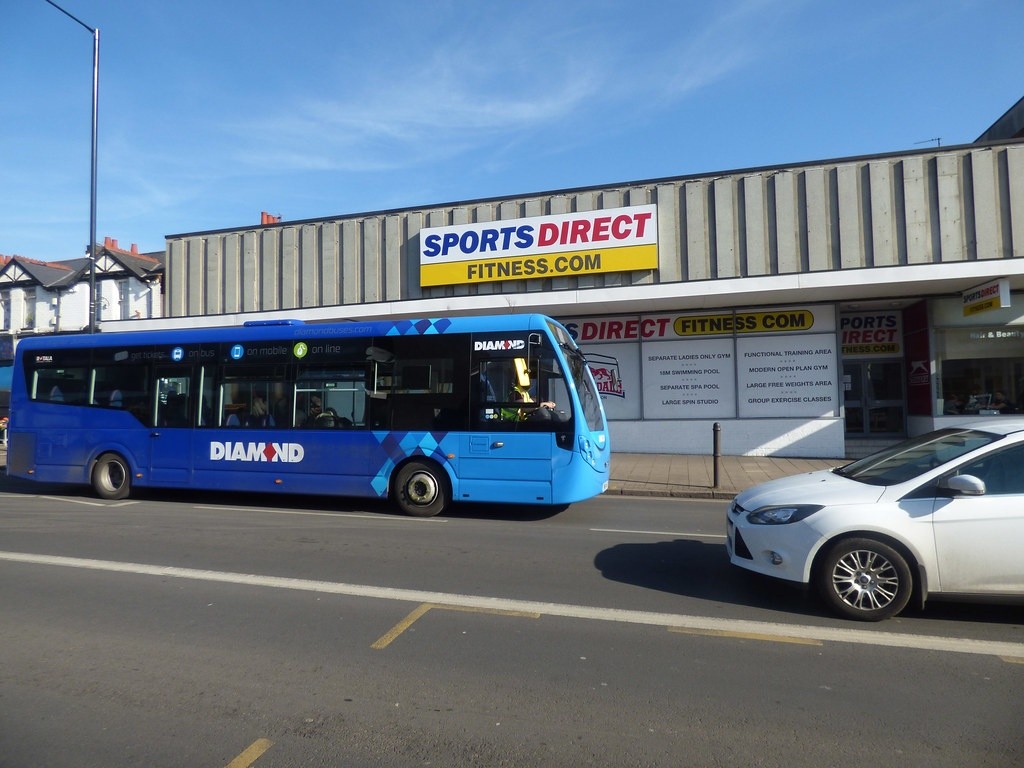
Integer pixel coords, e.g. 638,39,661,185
726,420,1024,622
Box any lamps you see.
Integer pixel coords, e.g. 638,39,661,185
969,393,992,409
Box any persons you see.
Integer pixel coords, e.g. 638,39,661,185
501,379,556,421
166,381,180,403
310,396,352,427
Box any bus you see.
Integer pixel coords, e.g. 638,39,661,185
6,319,626,518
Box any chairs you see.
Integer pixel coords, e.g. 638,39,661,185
50,383,277,427
